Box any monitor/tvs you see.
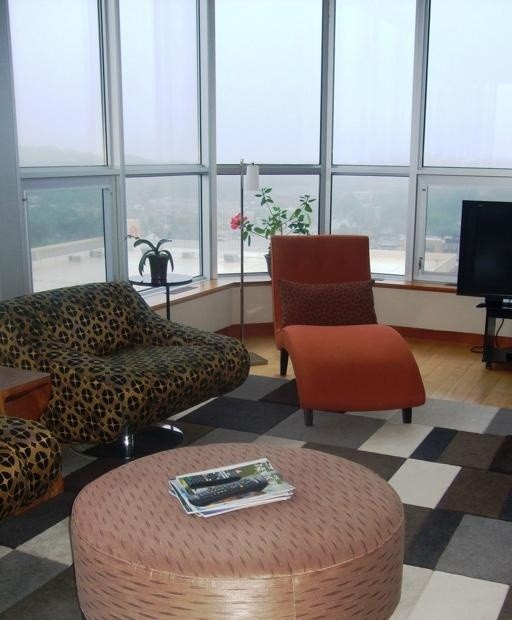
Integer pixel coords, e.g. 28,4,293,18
456,200,512,305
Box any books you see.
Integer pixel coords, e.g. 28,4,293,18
167,458,294,517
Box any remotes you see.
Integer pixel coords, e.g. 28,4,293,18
188,477,267,506
184,471,241,488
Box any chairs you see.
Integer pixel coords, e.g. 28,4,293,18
0,282,250,459
272,236,425,426
0,417,64,520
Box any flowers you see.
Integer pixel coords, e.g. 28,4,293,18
230,187,316,249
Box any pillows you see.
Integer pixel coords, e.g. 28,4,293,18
278,280,378,328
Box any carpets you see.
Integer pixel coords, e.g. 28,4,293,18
0,375,512,620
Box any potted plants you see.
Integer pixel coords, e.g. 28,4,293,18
128,234,173,280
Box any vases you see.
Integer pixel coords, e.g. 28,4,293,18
265,254,271,278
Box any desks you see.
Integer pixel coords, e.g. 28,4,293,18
476,303,512,368
0,367,52,422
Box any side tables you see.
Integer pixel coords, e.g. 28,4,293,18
129,274,192,320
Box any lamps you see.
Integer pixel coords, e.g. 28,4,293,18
240,159,267,365
